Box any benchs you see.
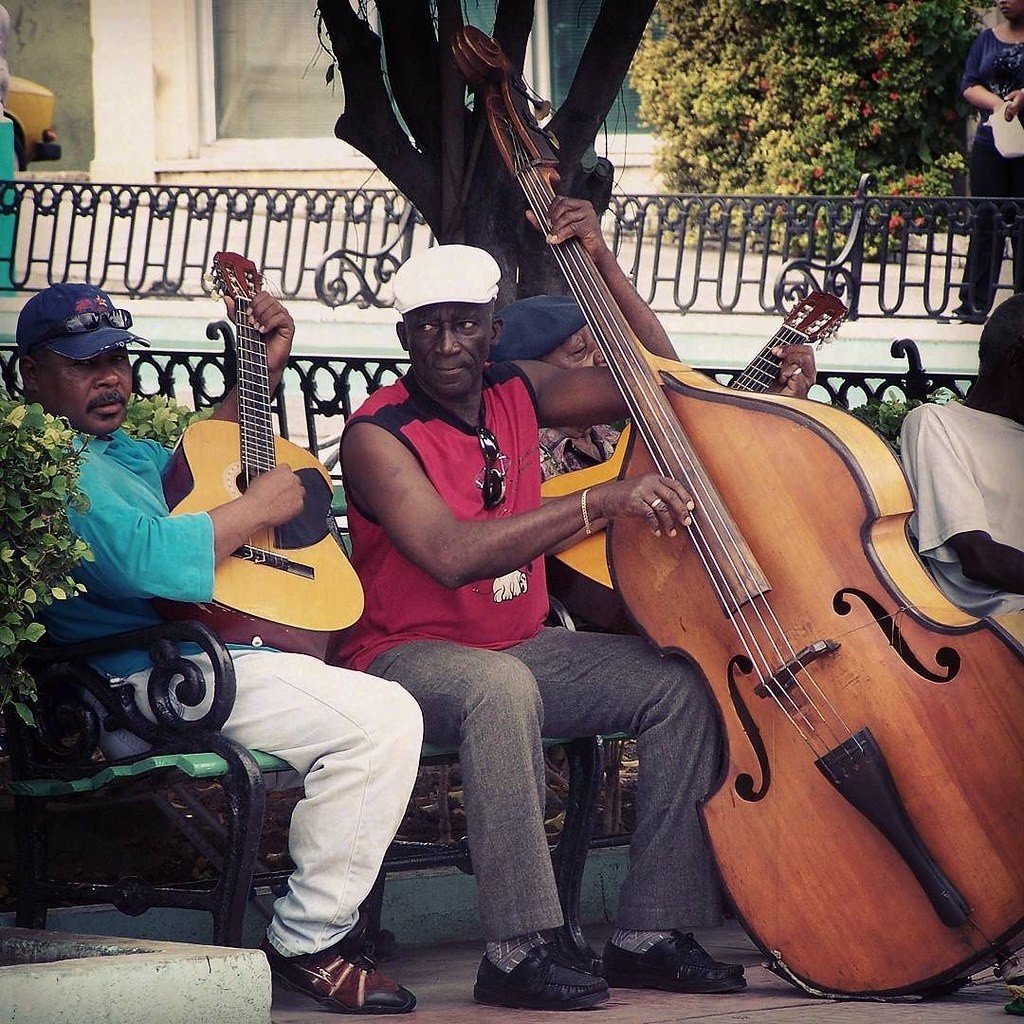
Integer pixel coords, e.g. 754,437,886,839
0,585,615,994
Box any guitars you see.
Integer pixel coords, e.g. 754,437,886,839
157,249,366,662
535,289,854,631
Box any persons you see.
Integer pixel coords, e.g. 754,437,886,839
332,193,744,1010
942,0,1024,321
481,293,822,632
897,293,1024,618
9,283,422,1017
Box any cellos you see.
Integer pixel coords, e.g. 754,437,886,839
445,22,1024,998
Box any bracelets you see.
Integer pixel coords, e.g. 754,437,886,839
580,486,595,536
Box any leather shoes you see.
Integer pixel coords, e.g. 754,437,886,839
473,950,612,1009
262,942,417,1012
605,934,746,995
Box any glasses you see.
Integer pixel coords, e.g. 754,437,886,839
474,426,509,507
27,310,134,348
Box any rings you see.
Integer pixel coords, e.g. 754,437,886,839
651,499,662,509
792,367,800,376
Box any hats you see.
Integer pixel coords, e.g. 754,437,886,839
491,295,586,360
16,283,152,359
393,244,501,311
